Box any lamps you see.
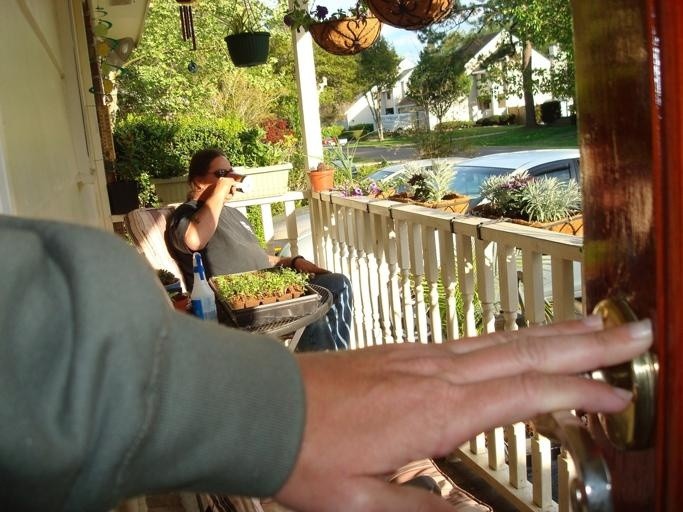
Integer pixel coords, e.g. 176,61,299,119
101,36,134,61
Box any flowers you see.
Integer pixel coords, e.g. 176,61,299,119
330,179,382,196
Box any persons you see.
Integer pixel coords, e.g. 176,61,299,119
164,150,354,353
0,215,653,511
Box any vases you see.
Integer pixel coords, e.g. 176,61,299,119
307,169,334,193
363,189,395,200
367,0,454,31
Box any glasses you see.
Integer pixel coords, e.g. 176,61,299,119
207,169,229,178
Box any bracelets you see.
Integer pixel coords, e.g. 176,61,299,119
290,256,303,266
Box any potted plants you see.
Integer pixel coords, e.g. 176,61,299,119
464,168,584,256
106,119,293,215
210,3,269,68
281,1,381,56
213,267,309,311
385,154,470,215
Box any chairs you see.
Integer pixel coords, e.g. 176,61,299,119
124,206,187,293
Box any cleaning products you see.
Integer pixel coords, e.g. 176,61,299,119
191,251,218,323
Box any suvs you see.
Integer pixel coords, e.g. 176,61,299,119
426,149,581,197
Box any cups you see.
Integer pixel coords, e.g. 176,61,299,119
226,172,253,193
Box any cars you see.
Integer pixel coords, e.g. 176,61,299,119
358,156,470,191
